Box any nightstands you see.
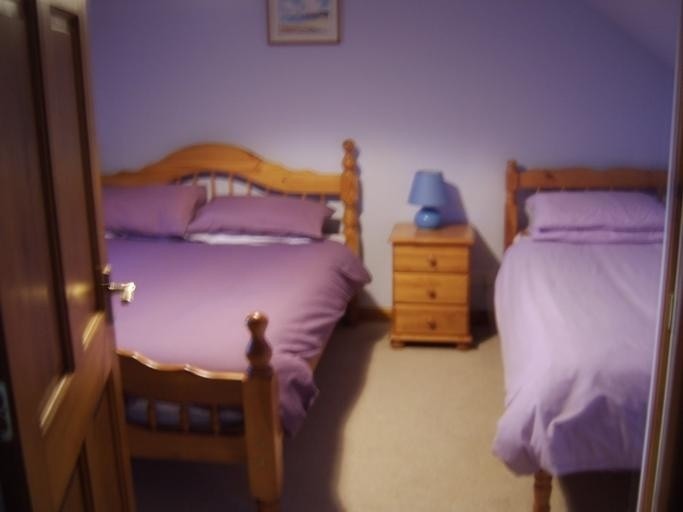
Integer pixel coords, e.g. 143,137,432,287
388,222,476,352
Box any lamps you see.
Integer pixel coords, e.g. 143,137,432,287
407,172,450,230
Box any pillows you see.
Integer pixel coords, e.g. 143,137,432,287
185,197,333,240
102,185,206,239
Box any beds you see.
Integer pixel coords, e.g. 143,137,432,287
102,138,372,512
493,160,683,512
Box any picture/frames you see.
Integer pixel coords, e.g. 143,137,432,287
268,0,343,44
